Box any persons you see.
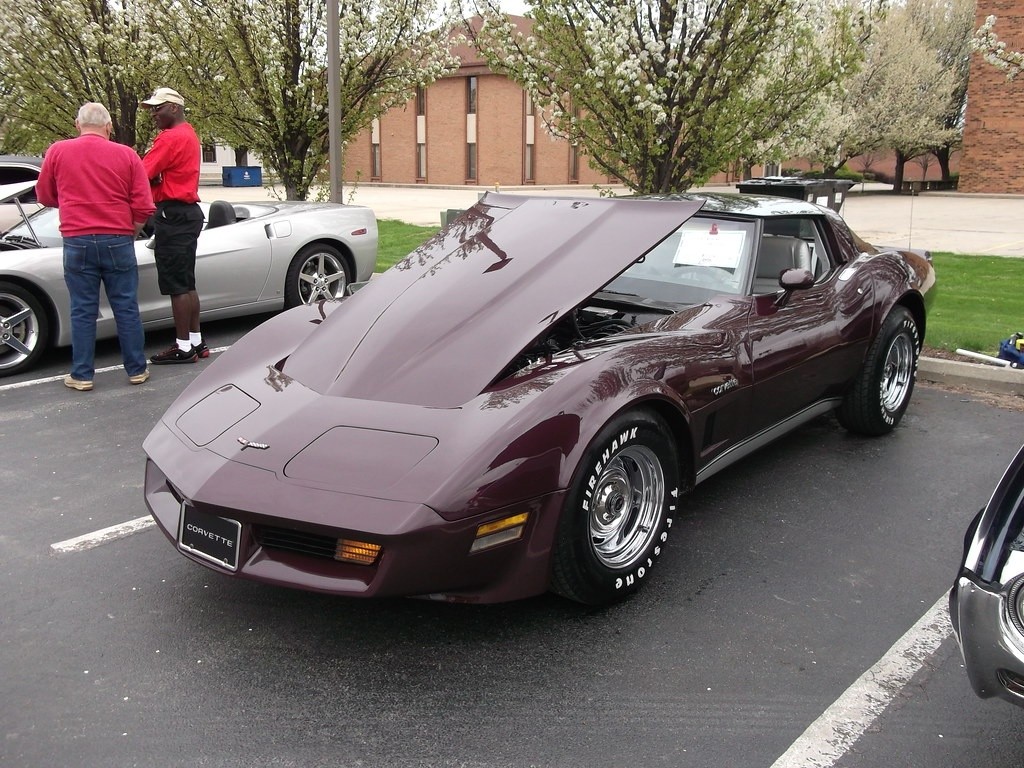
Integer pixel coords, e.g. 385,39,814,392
36,102,157,391
138,87,210,365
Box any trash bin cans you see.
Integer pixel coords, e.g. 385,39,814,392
736,177,855,214
222,166,262,187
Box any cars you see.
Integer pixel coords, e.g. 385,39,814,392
0,156,59,233
949,444,1024,711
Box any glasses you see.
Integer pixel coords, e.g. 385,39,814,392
150,103,179,113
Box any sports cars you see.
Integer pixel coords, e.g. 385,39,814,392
0,168,379,385
140,189,938,612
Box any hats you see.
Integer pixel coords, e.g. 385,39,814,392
140,87,185,108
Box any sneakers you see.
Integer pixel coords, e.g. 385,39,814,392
191,339,209,358
150,343,198,364
129,367,149,385
63,374,93,391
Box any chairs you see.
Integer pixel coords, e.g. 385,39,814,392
749,233,811,294
204,201,236,229
